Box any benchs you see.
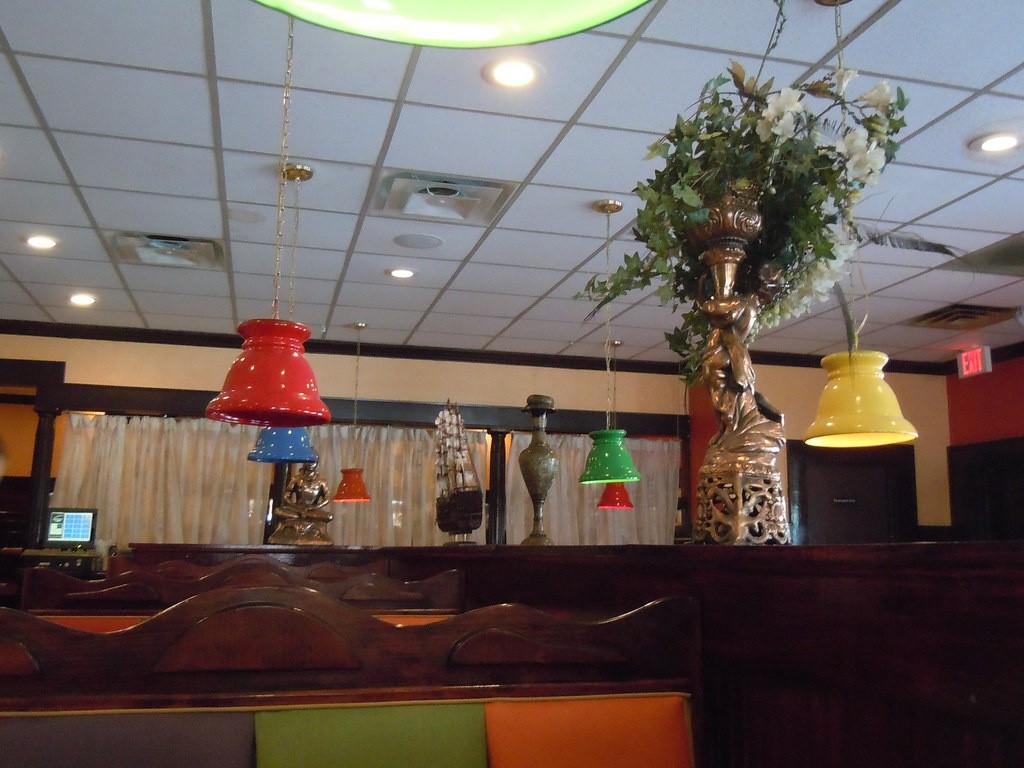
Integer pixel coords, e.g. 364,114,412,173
0,556,707,768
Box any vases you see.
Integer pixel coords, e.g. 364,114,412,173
518,395,559,546
675,178,791,546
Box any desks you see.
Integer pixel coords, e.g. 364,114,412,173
22,549,103,571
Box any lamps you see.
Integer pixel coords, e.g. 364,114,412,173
246,163,320,467
579,198,641,484
596,339,635,511
330,322,373,504
804,0,920,448
250,0,650,49
203,16,333,429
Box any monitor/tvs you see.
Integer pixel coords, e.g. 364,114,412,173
44,508,98,549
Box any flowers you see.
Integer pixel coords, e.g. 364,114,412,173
573,0,909,389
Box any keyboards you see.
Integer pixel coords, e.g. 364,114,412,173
22,550,96,557
